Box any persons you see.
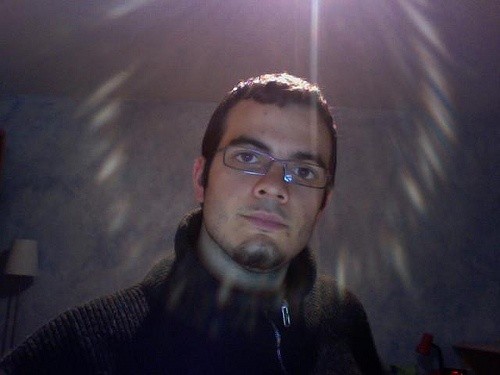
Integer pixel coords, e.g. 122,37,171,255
1,69,388,375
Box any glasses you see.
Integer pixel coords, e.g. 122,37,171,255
214,145,333,189
